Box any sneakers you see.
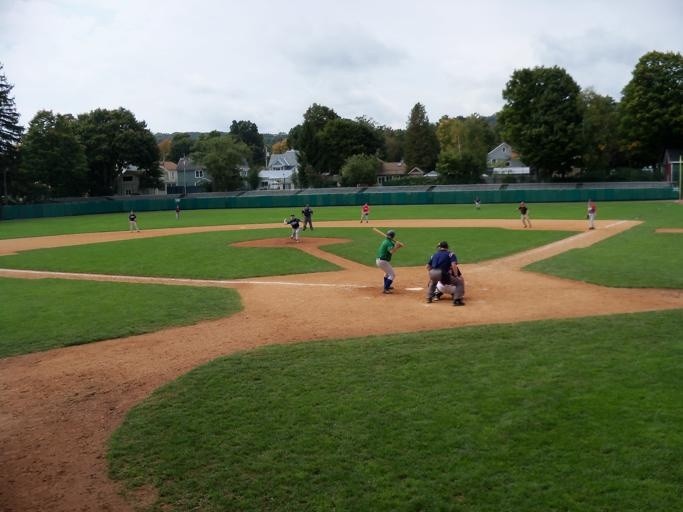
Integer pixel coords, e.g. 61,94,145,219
384,286,393,294
454,299,464,305
425,293,440,302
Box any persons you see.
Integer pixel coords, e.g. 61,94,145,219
375,230,403,294
474,193,481,208
360,202,369,223
513,201,533,228
128,209,139,232
588,199,596,229
283,215,304,242
302,203,314,230
176,204,181,220
433,265,464,301
424,241,466,305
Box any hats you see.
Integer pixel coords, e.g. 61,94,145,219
437,241,448,248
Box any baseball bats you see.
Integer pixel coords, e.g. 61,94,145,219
373,227,404,247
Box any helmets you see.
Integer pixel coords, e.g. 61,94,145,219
386,231,395,238
